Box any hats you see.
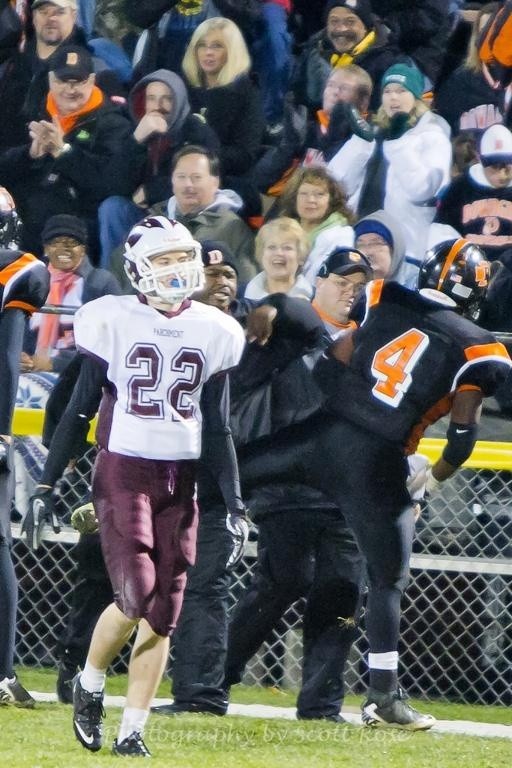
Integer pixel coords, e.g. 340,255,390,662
41,213,89,245
479,124,512,167
31,0,77,10
326,250,374,283
379,62,426,100
201,240,239,276
322,0,373,30
46,45,97,83
354,218,394,247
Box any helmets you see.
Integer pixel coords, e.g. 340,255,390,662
121,215,206,305
0,185,24,247
416,237,492,323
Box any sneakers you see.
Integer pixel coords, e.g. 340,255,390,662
57,659,78,704
70,670,107,752
360,687,436,732
0,674,37,709
110,730,153,760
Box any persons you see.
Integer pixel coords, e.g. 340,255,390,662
195,234,512,734
40,351,115,714
0,185,53,708
21,212,251,761
1,2,511,561
219,242,370,724
147,238,319,718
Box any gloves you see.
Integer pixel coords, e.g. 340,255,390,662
18,484,60,551
221,510,252,571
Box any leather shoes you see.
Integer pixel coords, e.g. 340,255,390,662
296,711,355,729
150,701,228,717
